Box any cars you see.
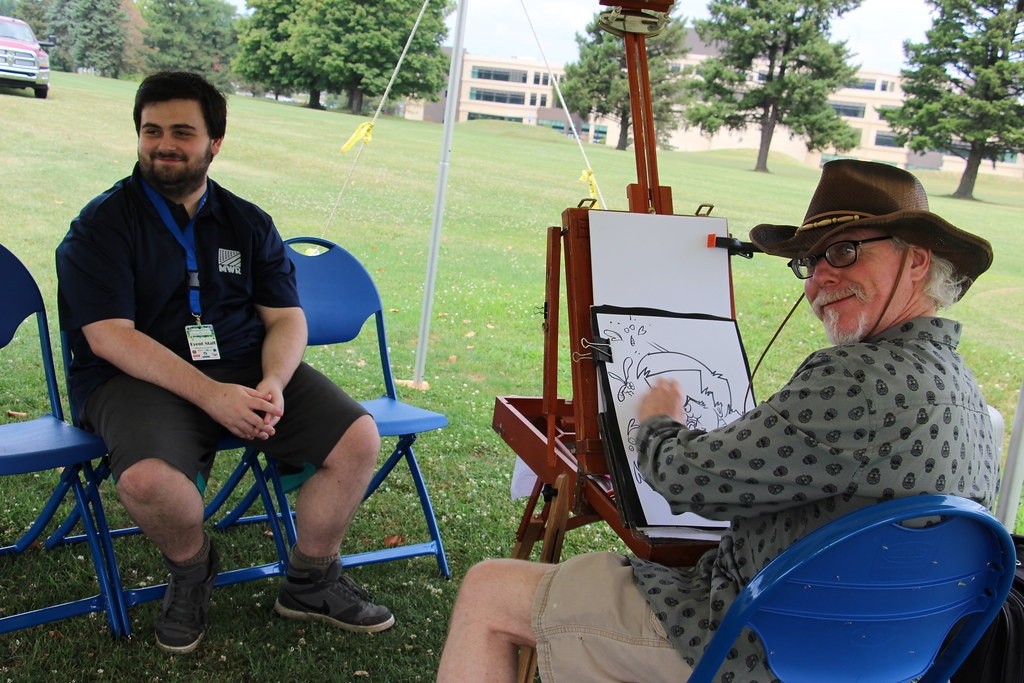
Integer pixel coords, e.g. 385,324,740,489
1,14,50,99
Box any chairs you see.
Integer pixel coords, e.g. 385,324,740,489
49,289,289,638
686,494,1015,683
0,244,123,638
211,236,453,582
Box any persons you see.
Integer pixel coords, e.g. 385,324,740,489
435,161,1002,682
55,71,396,655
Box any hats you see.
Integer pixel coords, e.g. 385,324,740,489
748,160,995,303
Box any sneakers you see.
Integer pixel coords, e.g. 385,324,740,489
273,560,395,633
154,540,221,654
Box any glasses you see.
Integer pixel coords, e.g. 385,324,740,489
787,236,894,279
